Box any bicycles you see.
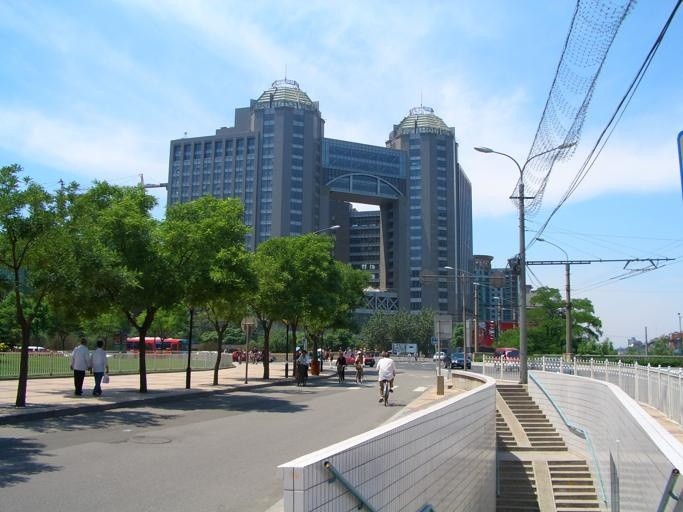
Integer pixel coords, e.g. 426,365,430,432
382,380,392,406
295,362,363,387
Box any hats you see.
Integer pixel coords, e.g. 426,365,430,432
356,351,363,355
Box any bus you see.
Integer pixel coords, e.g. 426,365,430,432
125,336,189,359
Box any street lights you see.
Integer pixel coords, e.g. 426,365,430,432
442,266,504,355
473,140,580,385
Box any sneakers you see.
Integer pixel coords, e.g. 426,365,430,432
75,389,84,396
379,396,383,402
93,390,102,396
390,387,393,393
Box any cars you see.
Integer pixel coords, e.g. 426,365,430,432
342,351,375,368
443,352,471,370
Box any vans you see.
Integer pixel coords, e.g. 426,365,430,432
492,346,520,372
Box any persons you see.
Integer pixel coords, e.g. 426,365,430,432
408,352,418,361
237,345,385,383
70,338,90,395
375,352,396,402
90,340,109,396
